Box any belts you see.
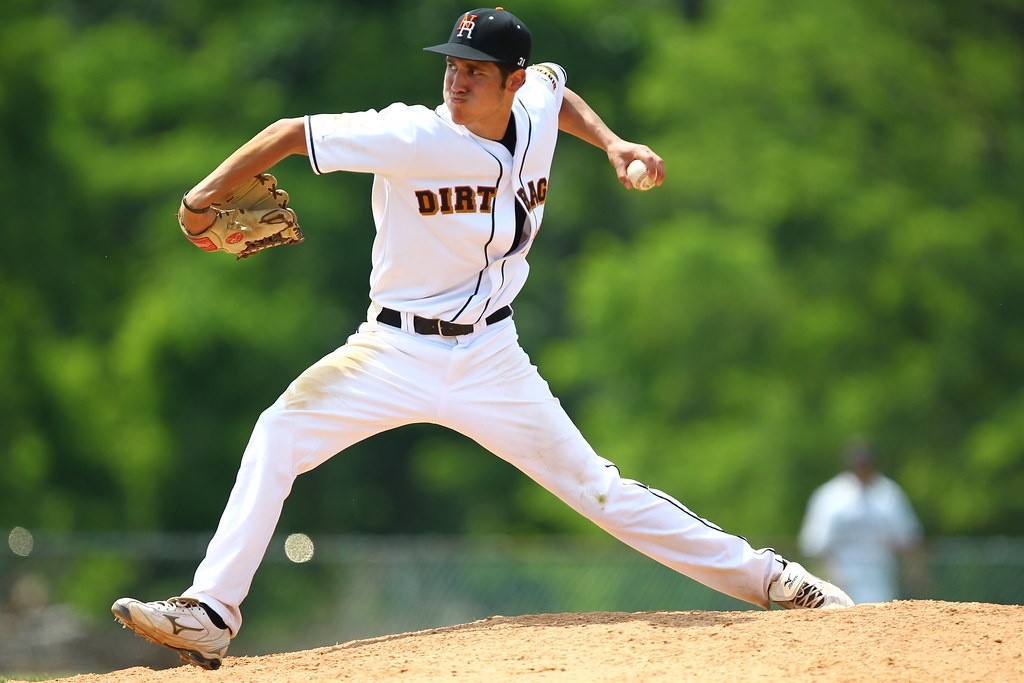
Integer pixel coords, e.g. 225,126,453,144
376,305,513,339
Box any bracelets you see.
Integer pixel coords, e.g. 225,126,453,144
183,191,211,214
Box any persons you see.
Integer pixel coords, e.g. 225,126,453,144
797,439,928,603
110,7,855,670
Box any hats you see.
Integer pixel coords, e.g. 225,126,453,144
422,6,533,74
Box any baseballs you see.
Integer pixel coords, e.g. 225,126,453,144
626,159,658,192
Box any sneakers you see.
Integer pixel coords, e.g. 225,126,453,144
768,558,855,610
111,597,232,672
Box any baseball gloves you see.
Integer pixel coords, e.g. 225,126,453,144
178,171,306,254
